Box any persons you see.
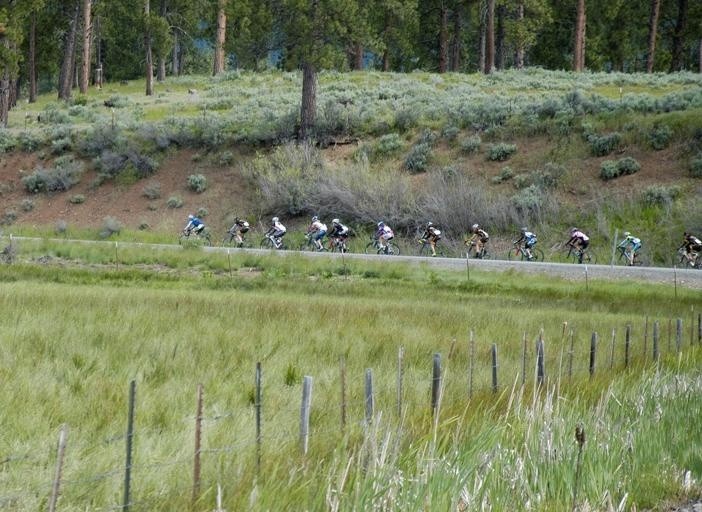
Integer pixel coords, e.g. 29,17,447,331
230,216,250,248
514,227,538,261
306,216,328,251
469,223,489,259
420,221,442,257
263,216,286,249
677,230,702,266
183,213,205,236
616,231,642,266
564,227,589,261
327,218,350,253
375,221,394,252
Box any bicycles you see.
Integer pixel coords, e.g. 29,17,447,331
222,230,255,249
609,245,649,267
508,240,544,262
179,228,211,247
413,237,448,258
559,242,597,264
260,232,293,250
460,240,496,260
321,234,355,253
672,248,702,270
300,232,333,253
365,236,400,255
